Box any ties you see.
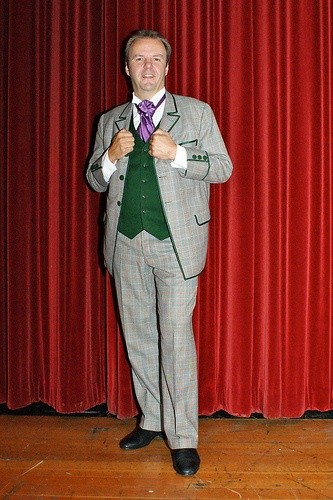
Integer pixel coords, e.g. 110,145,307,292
135,100,156,142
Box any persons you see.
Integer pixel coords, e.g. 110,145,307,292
84,29,235,478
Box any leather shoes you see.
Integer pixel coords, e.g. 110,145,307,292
169,447,200,477
118,425,163,450
